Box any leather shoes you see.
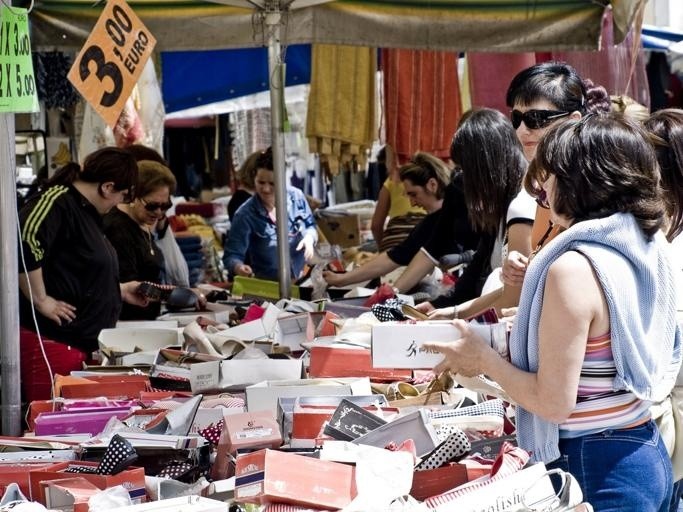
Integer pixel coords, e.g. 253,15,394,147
368,372,454,402
136,277,269,357
413,428,473,474
299,315,368,356
372,290,433,324
0,366,247,512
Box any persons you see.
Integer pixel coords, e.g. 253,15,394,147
17,145,206,404
322,61,683,511
222,146,320,284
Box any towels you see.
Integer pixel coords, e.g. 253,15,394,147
308,44,534,158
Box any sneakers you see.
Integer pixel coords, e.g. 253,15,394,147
423,445,593,512
424,397,505,431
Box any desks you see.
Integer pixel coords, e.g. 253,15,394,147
0,242,599,512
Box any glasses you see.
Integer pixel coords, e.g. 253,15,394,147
140,197,172,212
510,108,569,130
534,189,551,210
